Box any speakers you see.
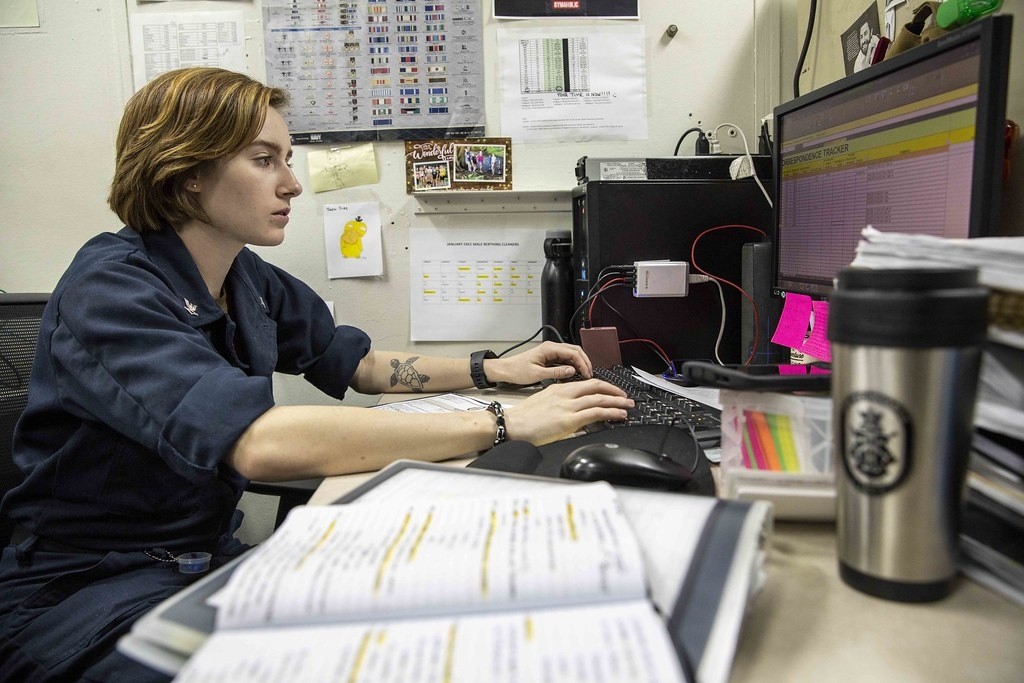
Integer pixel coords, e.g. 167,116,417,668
739,241,783,366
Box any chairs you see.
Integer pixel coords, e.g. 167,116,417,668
0,291,317,534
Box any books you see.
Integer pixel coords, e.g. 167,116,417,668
111,458,788,683
850,222,1024,610
718,387,834,521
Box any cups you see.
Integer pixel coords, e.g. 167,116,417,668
824,264,991,604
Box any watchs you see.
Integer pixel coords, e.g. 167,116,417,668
469,349,499,390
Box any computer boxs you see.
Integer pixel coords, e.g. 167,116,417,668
570,177,767,375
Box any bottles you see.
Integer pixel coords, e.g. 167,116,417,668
541,228,576,346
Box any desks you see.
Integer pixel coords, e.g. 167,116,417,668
307,387,1024,682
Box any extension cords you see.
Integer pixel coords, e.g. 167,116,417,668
579,151,773,181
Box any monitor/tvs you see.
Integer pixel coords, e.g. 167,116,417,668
766,8,1013,302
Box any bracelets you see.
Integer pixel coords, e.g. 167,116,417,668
486,400,507,449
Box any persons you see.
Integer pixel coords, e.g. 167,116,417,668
0,67,635,683
854,12,881,74
417,165,446,188
465,147,502,175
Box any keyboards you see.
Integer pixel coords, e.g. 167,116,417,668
538,365,723,452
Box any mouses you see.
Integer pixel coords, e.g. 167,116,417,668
559,443,695,496
